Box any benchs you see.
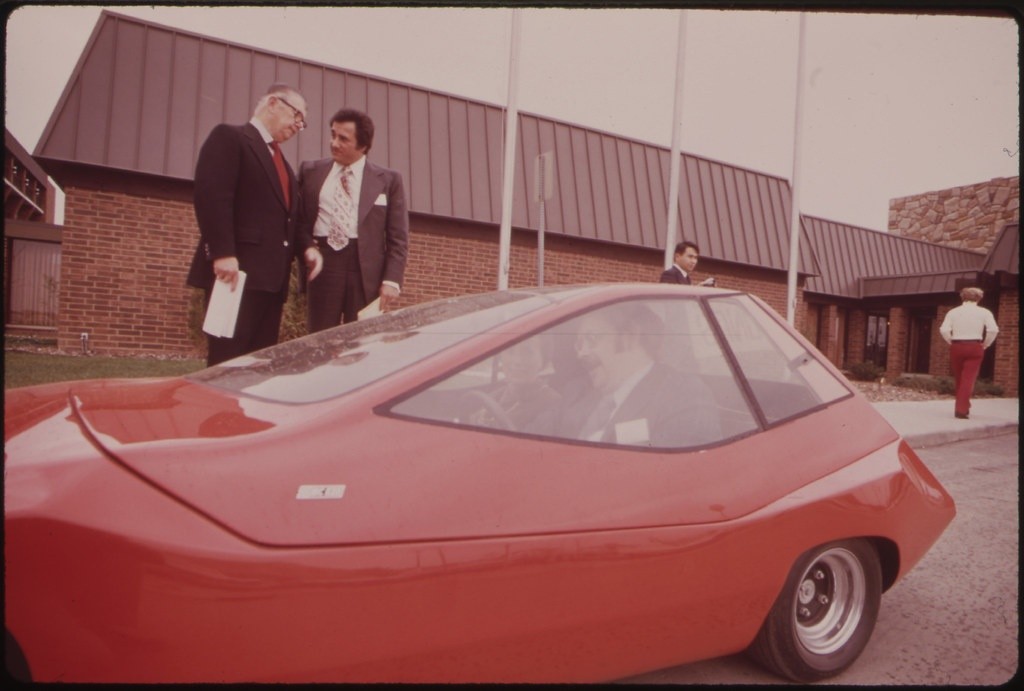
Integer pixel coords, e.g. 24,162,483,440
696,375,816,444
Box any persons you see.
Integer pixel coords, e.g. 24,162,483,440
185,85,322,369
938,287,999,418
300,108,408,335
661,241,717,287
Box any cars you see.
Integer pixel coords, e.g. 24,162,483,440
0,283,961,686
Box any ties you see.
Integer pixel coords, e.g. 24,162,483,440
327,167,351,251
268,141,290,211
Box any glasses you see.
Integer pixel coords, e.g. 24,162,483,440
278,96,308,130
